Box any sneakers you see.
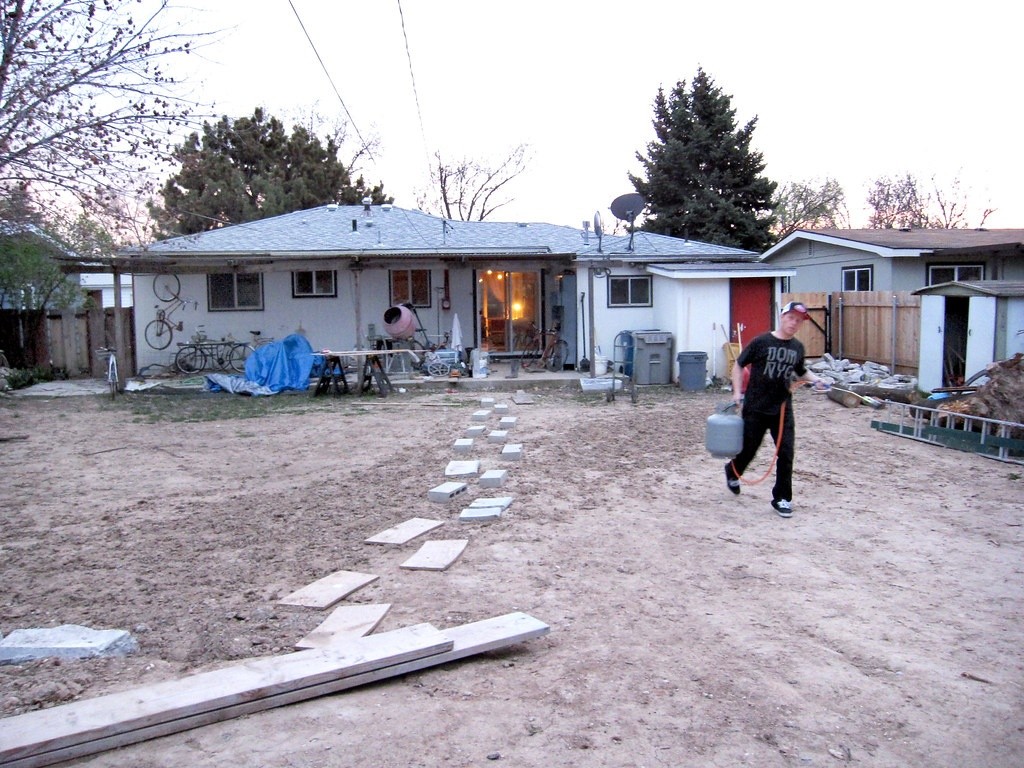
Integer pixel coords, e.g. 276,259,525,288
724,461,740,495
770,499,793,517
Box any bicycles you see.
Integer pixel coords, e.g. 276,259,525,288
145,271,199,350
521,321,570,373
407,328,453,377
95,346,125,401
176,325,276,375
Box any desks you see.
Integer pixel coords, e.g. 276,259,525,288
310,350,433,397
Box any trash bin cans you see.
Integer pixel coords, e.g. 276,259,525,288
676,351,708,392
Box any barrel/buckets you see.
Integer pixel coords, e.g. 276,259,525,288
468,348,489,379
677,351,707,391
381,306,417,340
594,355,608,375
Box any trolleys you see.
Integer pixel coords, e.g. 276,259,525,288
605,330,639,404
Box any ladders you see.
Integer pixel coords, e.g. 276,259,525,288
869,400,1024,465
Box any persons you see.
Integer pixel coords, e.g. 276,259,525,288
724,300,832,519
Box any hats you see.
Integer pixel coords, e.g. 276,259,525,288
780,301,809,320
